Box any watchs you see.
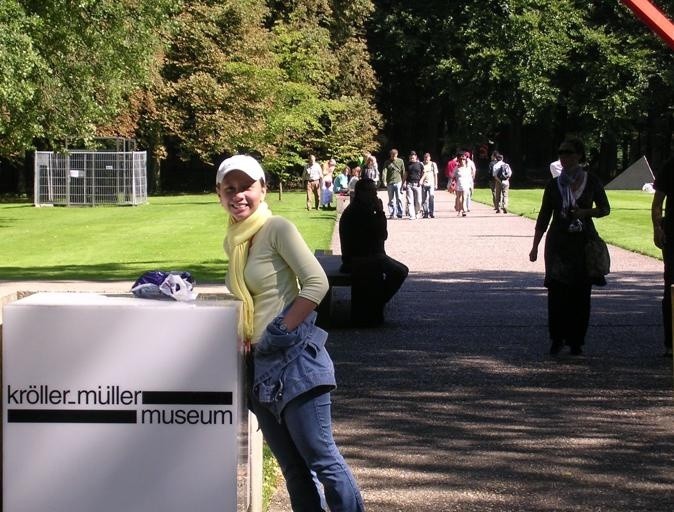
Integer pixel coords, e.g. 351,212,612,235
281,320,290,332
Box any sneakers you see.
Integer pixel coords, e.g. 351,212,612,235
410,212,466,220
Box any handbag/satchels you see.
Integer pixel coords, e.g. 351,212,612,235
585,233,610,277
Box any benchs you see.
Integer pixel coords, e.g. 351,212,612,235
314,255,364,327
336,193,351,214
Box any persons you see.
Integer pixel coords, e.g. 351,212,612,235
213,155,367,511
529,137,611,356
339,179,408,323
301,147,512,219
650,132,673,359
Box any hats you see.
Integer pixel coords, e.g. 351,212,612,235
216,155,265,184
465,152,470,157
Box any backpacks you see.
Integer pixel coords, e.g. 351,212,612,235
497,164,509,180
